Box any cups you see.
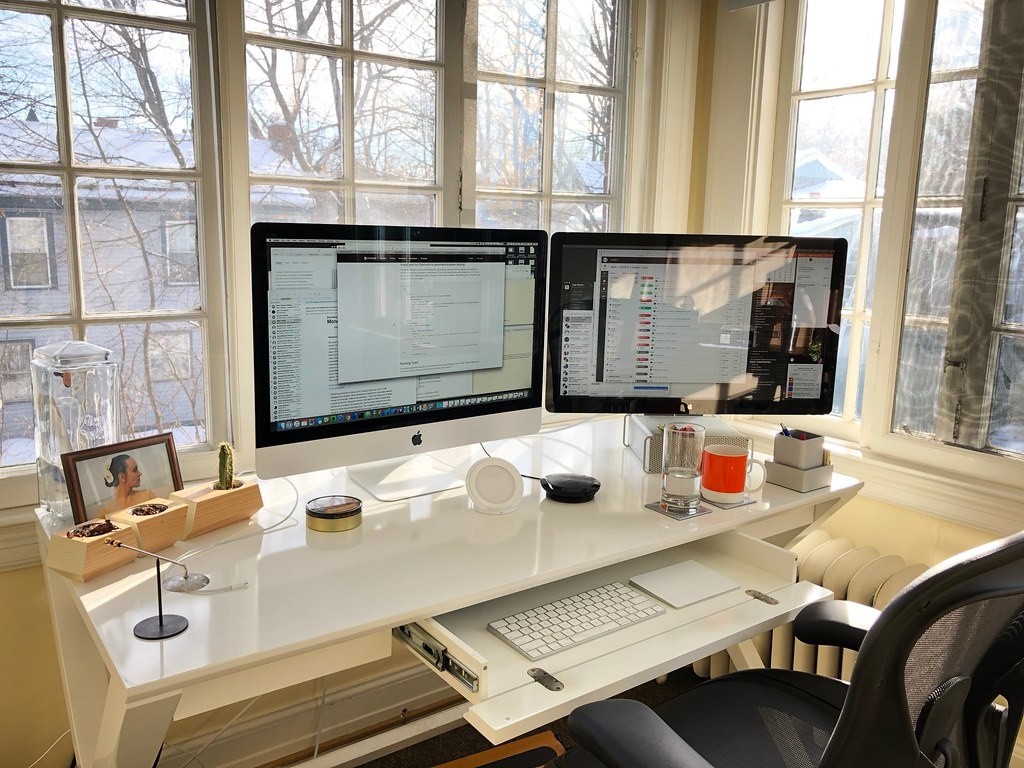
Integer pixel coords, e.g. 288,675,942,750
701,444,767,503
661,423,706,515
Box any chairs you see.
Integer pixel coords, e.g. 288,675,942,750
540,525,1024,767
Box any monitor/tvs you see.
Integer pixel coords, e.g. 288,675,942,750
250,222,549,501
545,232,849,415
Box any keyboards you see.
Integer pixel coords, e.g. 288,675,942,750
487,581,670,662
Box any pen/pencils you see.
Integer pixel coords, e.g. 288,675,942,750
780,423,792,437
800,434,807,440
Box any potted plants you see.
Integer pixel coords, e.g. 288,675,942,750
166,441,264,542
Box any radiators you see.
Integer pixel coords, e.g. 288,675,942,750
647,532,936,687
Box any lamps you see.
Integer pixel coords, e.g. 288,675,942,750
103,535,211,640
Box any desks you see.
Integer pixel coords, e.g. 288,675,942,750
35,416,863,768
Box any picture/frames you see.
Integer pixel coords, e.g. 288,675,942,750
59,432,187,526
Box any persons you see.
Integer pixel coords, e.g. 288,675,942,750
99,455,156,519
